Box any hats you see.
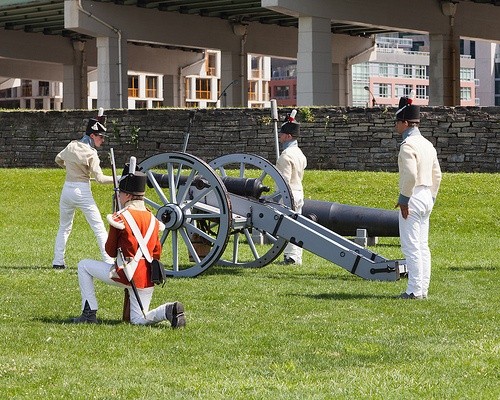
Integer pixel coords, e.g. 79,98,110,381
391,96,420,123
119,163,147,196
86,115,109,137
279,113,300,137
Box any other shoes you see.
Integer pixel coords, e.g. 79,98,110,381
394,292,427,300
53,265,65,269
165,302,186,329
275,258,295,266
72,311,97,324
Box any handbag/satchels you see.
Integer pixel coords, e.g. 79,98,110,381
151,259,166,284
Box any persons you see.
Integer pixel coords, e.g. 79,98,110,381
71,173,186,330
393,90,442,300
53,106,122,271
275,110,307,266
